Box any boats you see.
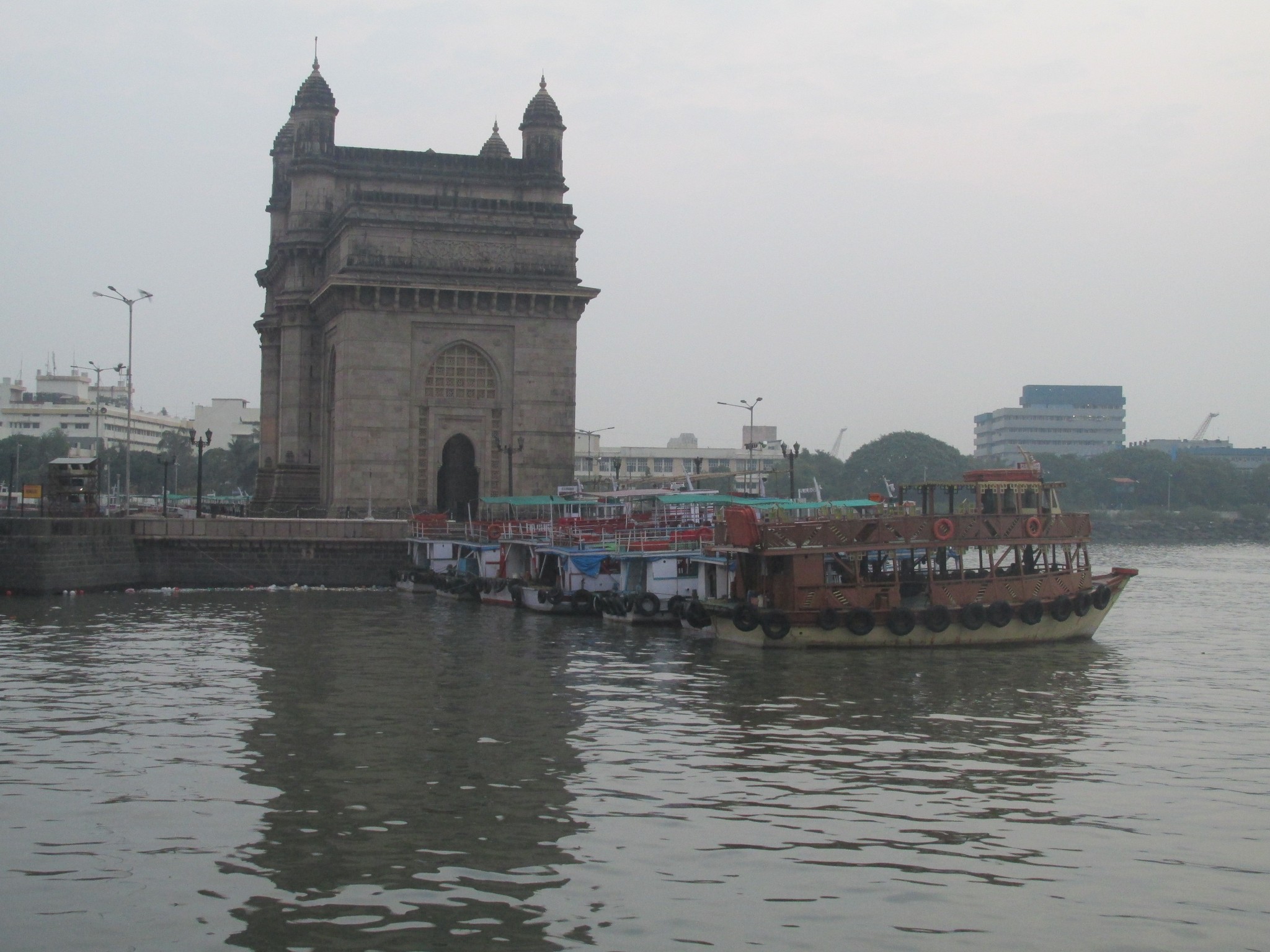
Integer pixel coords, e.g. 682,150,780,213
394,446,1138,652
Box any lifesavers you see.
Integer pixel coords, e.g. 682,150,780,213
1026,517,1041,537
696,527,713,543
487,524,501,540
934,519,954,540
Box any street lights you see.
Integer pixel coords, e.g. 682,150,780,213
612,455,622,481
781,441,800,498
158,454,176,518
717,396,767,497
189,427,213,516
494,436,525,521
575,426,615,480
17,444,23,493
69,362,127,494
1165,470,1173,512
92,285,153,517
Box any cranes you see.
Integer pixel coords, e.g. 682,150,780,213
829,427,846,458
1191,411,1219,441
